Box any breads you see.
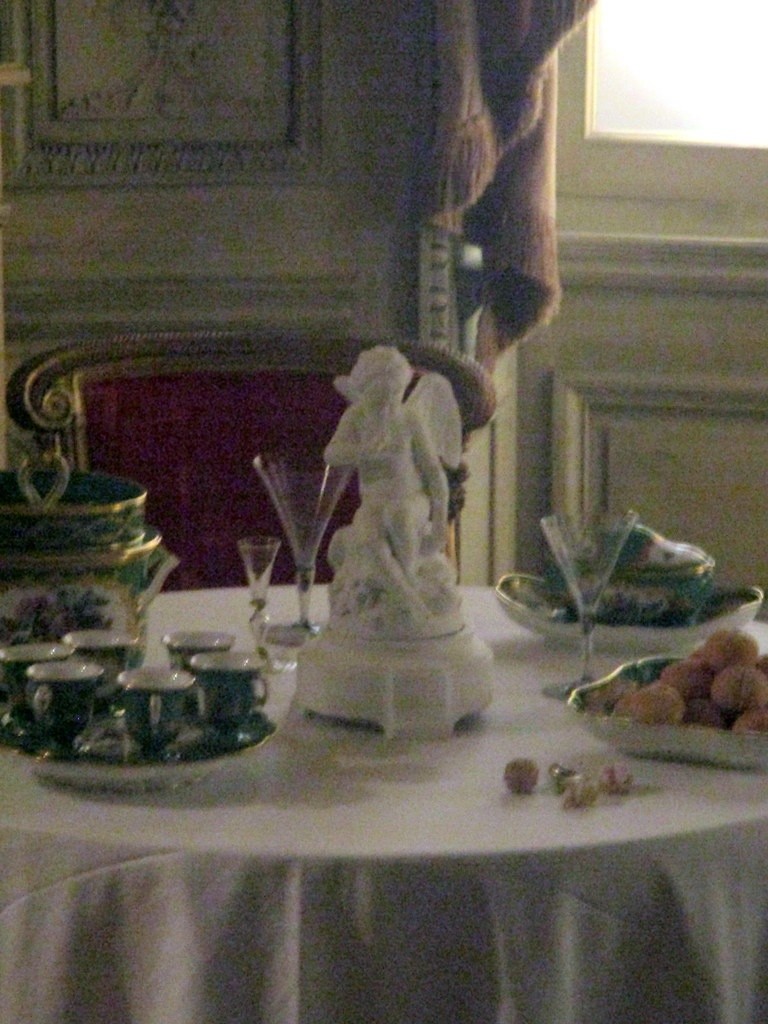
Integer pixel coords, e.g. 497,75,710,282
610,626,768,740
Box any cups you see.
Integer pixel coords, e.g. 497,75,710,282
1,630,271,756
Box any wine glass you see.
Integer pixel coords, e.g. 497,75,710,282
236,449,352,675
539,509,638,701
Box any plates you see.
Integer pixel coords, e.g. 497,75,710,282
33,713,278,793
496,574,763,654
566,656,766,770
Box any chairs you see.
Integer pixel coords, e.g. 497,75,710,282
5,332,497,593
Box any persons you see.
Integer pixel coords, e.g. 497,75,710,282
322,345,451,621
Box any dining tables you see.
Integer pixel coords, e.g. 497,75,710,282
0,584,768,1024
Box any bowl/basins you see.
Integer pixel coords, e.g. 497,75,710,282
550,511,718,628
